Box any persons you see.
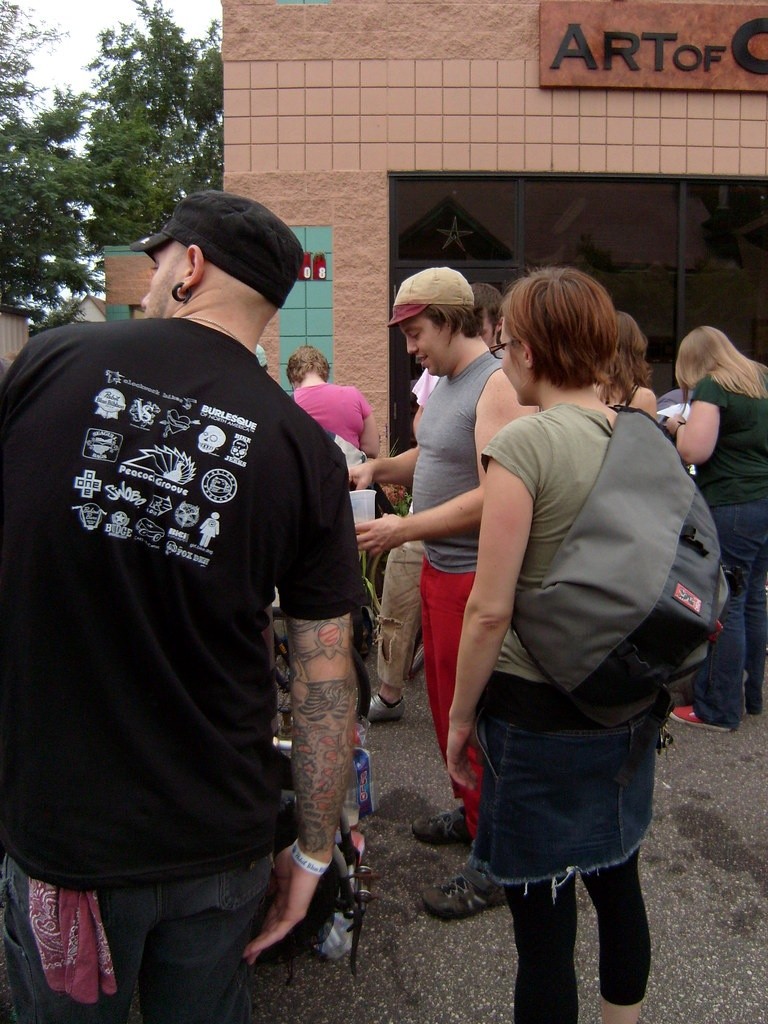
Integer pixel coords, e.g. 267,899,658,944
0,190,368,1024
447,268,661,1024
254,266,768,920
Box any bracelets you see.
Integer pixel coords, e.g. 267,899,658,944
291,838,333,874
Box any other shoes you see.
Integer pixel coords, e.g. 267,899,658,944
748,706,762,714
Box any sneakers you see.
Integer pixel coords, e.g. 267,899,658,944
423,875,508,920
366,694,406,722
669,704,733,732
412,805,471,844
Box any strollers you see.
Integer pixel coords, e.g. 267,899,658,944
271,735,376,971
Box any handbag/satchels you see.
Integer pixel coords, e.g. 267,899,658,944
511,405,732,726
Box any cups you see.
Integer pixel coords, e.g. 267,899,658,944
350,489,377,534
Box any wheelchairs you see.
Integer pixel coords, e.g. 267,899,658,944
364,460,425,678
272,598,375,731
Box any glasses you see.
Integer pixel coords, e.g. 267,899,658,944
489,339,523,359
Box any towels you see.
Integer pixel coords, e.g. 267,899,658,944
27,879,118,1006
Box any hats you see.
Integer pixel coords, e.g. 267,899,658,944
129,190,305,309
387,267,475,327
